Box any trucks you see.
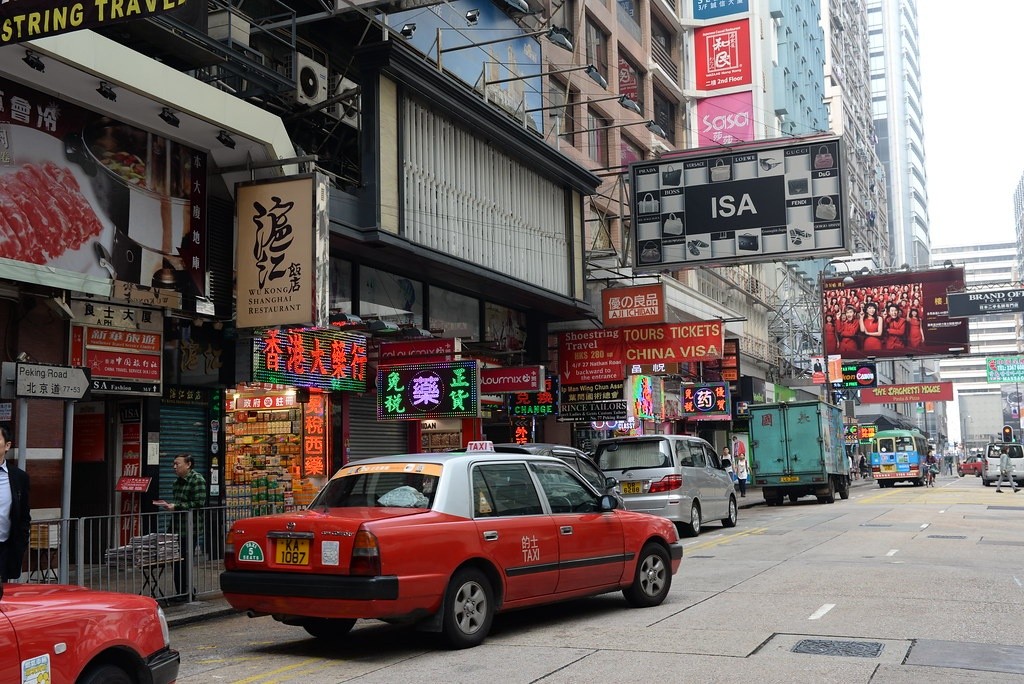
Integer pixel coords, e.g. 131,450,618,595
747,399,855,506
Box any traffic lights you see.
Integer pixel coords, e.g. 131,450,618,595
1001,425,1012,444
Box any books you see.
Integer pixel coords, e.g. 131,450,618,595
153,500,168,507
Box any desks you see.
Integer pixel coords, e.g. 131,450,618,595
25,546,62,584
111,557,185,609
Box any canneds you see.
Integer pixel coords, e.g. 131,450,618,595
243,421,290,435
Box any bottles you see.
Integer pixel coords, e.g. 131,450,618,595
252,475,285,516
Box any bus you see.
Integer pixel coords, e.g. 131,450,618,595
870,428,931,488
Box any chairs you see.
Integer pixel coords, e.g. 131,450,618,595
502,479,530,508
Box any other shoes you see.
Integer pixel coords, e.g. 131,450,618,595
1014,488,1021,492
741,491,746,497
996,490,1004,493
168,591,196,605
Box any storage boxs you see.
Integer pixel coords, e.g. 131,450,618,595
225,409,319,531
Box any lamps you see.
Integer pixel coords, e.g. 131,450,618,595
22,49,46,73
329,307,363,328
398,324,434,340
216,130,236,150
554,116,666,152
158,107,180,128
382,0,417,41
440,0,481,26
43,289,77,320
341,316,402,334
521,90,642,130
482,61,607,104
96,81,117,103
436,27,574,73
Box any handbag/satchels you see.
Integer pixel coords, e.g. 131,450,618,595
929,463,940,474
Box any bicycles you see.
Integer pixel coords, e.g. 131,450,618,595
923,462,937,488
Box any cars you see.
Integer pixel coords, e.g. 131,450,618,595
219,439,683,651
977,442,1024,487
0,582,181,684
440,442,626,513
957,455,983,477
594,435,739,538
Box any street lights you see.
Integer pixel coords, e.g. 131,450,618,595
821,260,858,403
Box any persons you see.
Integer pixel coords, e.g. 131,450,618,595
907,307,925,347
825,315,839,348
836,307,859,348
0,426,32,601
996,446,1021,493
944,453,953,475
822,283,924,321
738,452,748,497
814,359,822,372
859,303,883,349
883,304,906,349
859,456,867,477
163,452,207,602
733,436,745,466
720,447,735,482
923,450,936,487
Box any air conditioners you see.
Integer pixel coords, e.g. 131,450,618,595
327,74,363,130
281,52,328,113
